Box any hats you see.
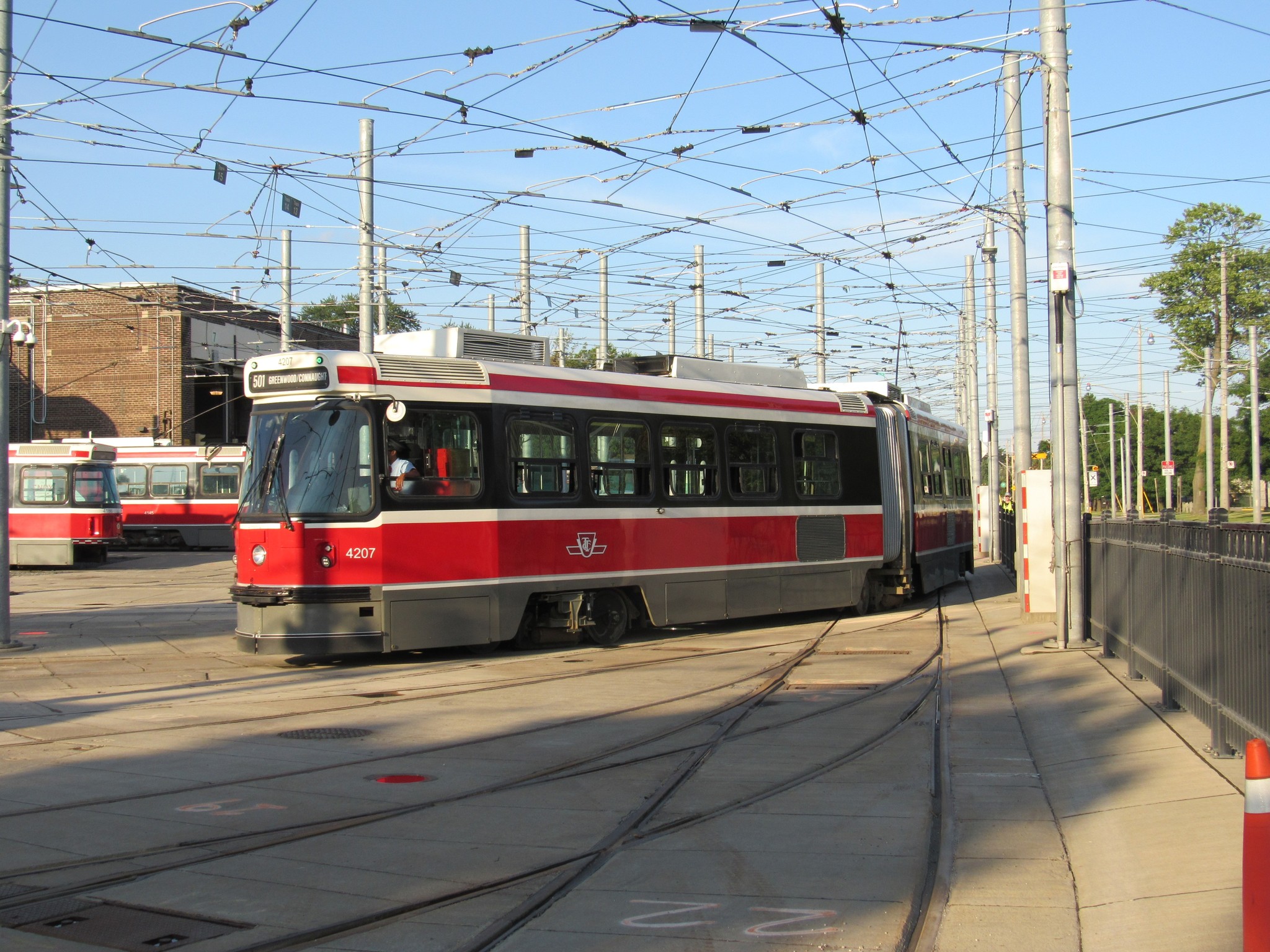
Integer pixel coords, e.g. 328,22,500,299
1005,492,1010,497
387,439,404,453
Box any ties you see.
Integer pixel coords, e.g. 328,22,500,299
389,466,392,476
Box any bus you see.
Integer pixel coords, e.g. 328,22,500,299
224,325,973,668
8,429,248,571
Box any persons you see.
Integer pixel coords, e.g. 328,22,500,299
387,436,420,495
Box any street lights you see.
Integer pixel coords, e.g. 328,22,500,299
1086,382,1132,512
1147,332,1214,522
